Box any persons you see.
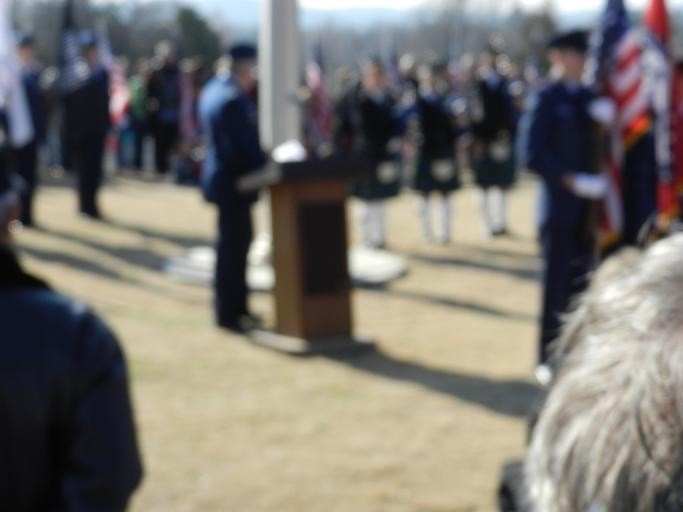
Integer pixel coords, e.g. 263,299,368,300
501,233,683,512
298,41,545,247
197,44,282,332
2,110,142,511
516,25,612,387
0,31,213,227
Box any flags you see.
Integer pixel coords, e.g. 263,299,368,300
588,0,683,234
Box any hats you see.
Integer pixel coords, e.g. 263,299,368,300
547,28,588,50
230,44,259,60
